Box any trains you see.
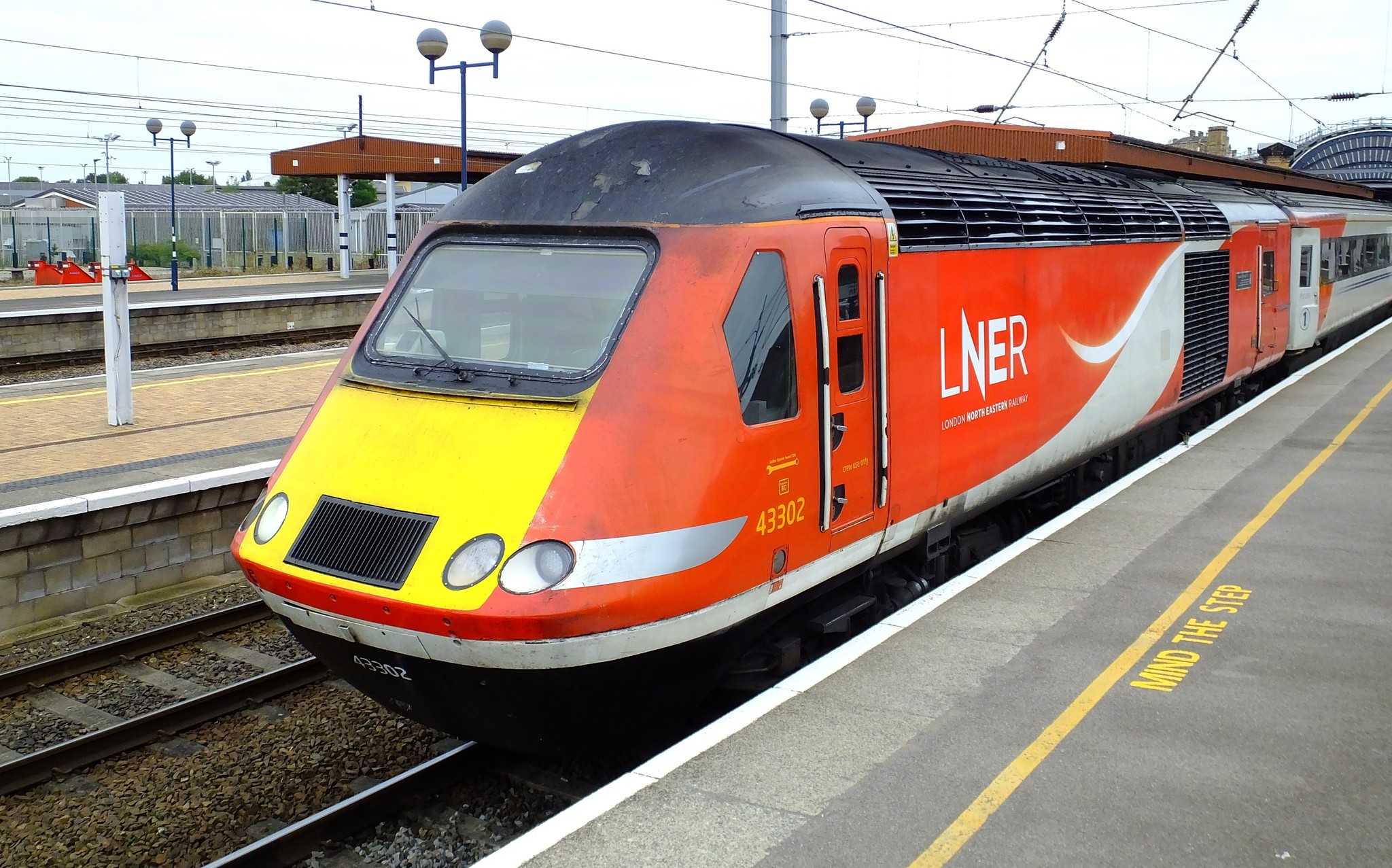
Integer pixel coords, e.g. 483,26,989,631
228,120,1391,743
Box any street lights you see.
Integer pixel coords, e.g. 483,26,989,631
36,166,46,191
2,156,12,204
92,133,121,192
416,20,512,195
810,97,876,140
186,168,195,184
336,123,358,138
141,171,149,184
146,119,196,291
207,160,222,191
93,159,102,250
79,163,89,189
